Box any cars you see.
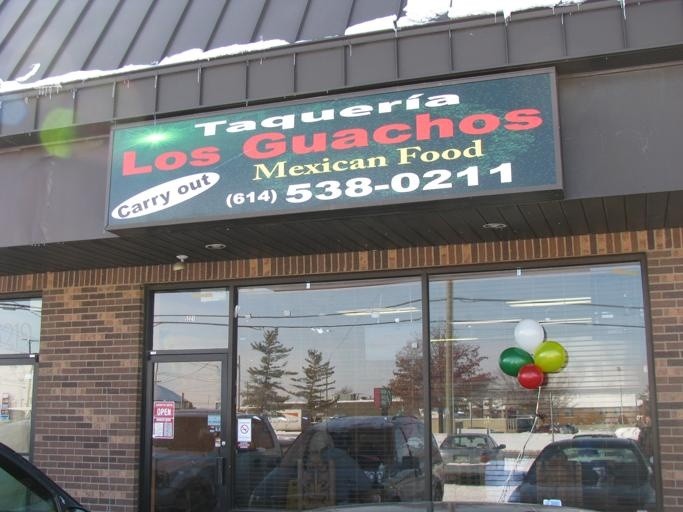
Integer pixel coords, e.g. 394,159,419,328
0,442,91,512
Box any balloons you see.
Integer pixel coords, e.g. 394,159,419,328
531,341,566,374
513,319,545,355
498,347,533,376
516,364,545,388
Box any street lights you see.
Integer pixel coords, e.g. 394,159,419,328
616,366,624,425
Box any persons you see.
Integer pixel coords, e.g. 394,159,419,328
637,415,653,470
304,431,375,508
